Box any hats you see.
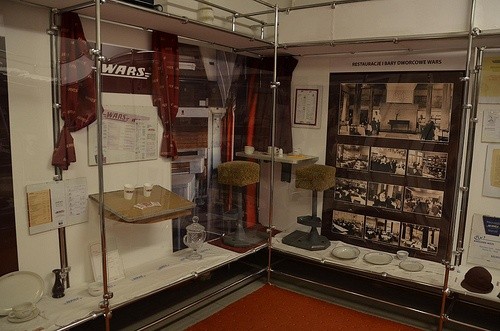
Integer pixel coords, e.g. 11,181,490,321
461,266,494,293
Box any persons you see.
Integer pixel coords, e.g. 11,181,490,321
334,112,446,251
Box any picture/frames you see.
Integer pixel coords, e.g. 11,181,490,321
292,86,323,129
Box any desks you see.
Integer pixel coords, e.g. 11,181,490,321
89,185,197,225
236,152,319,183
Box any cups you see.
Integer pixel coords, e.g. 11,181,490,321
143,182,154,197
396,250,409,259
267,146,273,154
88,281,104,296
244,146,255,155
8,303,33,318
123,184,135,200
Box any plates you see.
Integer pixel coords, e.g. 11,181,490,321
363,252,393,265
0,271,45,314
399,259,424,271
331,245,360,260
9,308,40,323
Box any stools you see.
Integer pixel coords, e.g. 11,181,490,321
218,161,266,247
282,165,336,251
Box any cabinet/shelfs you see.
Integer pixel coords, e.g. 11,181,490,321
321,70,470,263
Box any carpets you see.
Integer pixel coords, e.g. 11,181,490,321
183,283,426,331
